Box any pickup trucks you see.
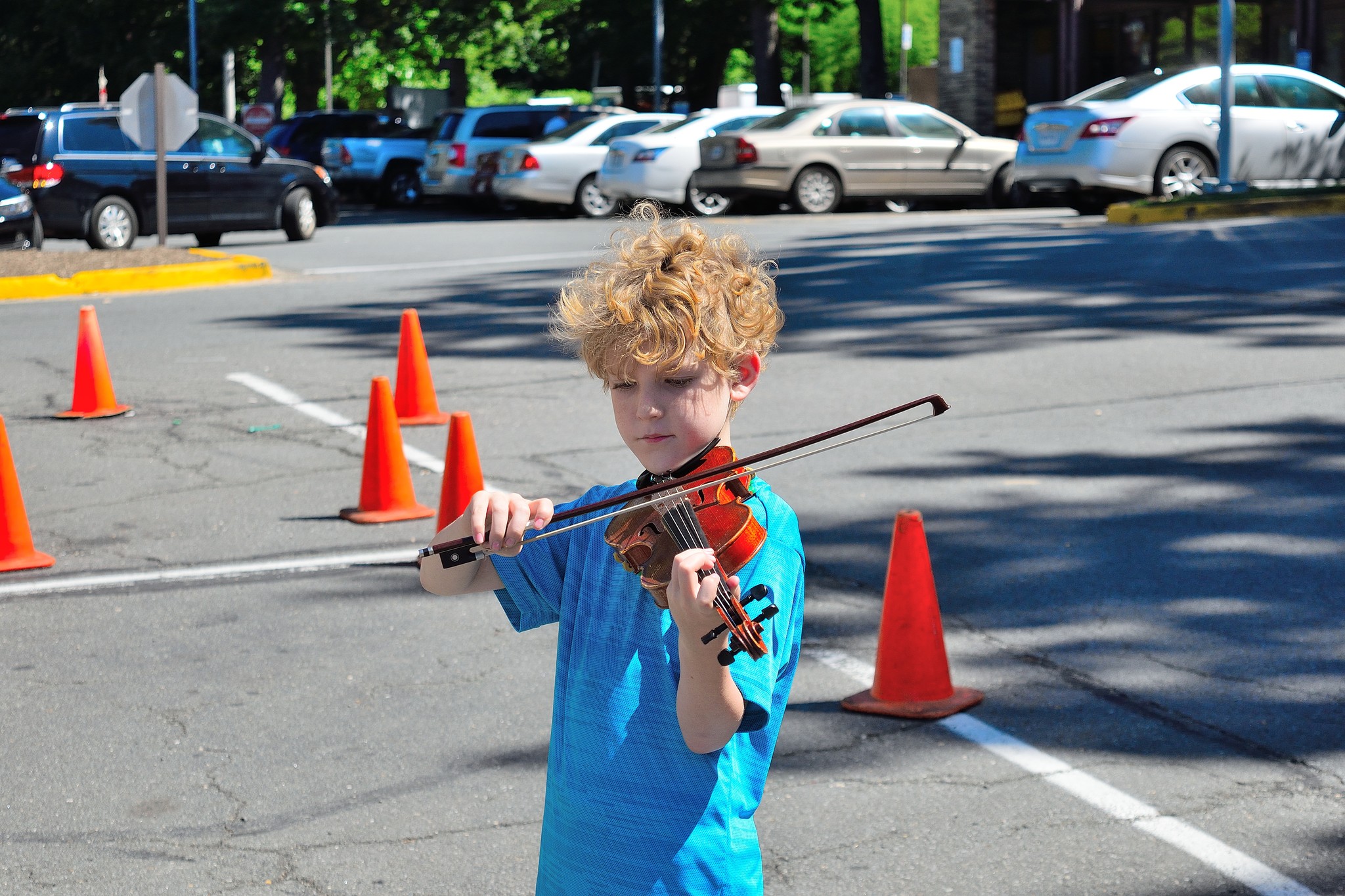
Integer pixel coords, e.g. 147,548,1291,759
322,101,531,212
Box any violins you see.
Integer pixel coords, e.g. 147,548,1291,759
604,445,780,667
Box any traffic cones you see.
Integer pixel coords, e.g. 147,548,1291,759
337,375,437,526
52,306,134,419
0,414,56,573
417,411,485,567
837,508,987,721
391,306,450,427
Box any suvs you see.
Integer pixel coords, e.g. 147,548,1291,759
261,109,415,204
414,96,640,219
0,98,340,251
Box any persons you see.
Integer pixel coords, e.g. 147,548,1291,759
418,200,807,896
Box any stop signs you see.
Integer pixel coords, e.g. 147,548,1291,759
241,102,276,137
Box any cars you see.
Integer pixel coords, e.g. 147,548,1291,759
689,98,1066,214
596,103,922,219
1014,60,1345,217
486,111,689,219
